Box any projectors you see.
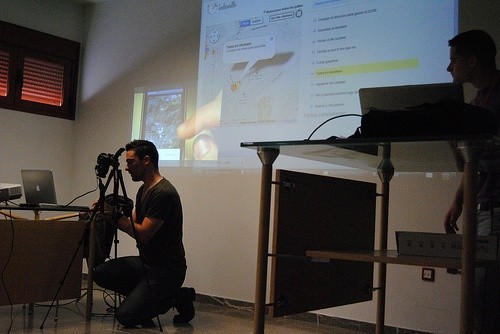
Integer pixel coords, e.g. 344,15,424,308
0,183,23,203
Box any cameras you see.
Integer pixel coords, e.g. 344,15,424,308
95,152,114,178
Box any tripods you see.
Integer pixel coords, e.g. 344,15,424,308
40,148,163,332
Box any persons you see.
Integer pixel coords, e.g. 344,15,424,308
443,29,500,334
91,140,196,329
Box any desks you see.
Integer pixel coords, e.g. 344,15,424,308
0,204,97,325
238,121,500,333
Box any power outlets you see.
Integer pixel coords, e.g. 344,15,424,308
426,268,435,280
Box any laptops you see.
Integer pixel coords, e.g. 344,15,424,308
359,83,464,118
20,168,57,204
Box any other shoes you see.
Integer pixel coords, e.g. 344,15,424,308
173,287,197,327
